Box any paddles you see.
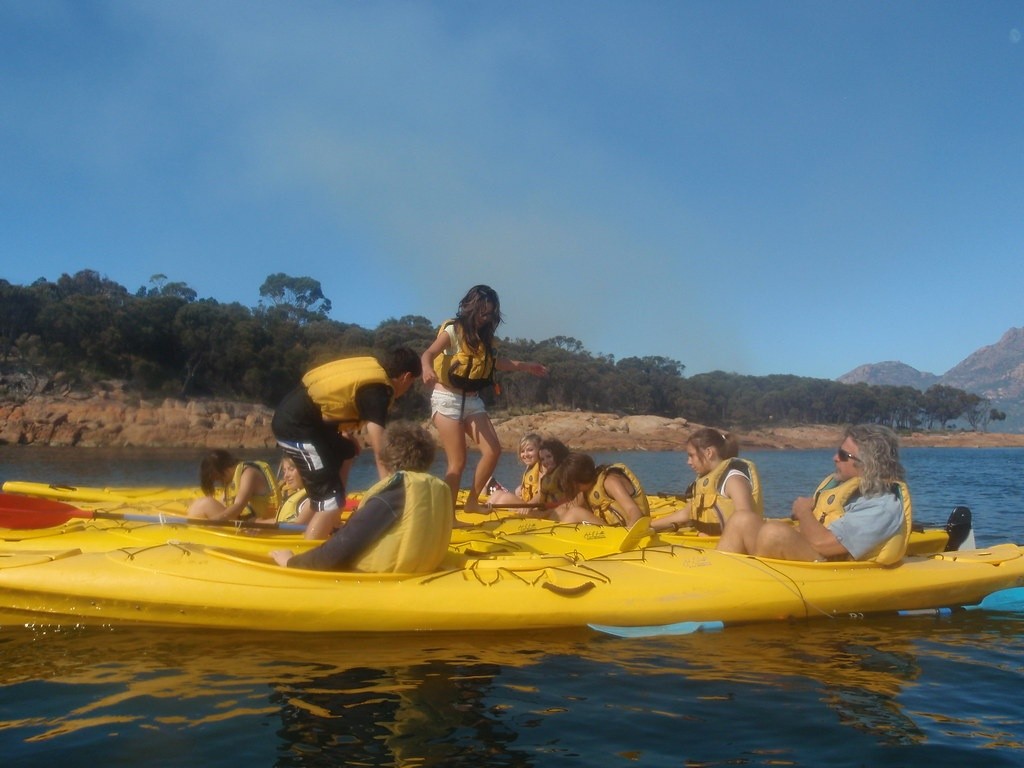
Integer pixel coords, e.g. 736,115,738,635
618,516,692,552
1,491,308,532
343,496,546,513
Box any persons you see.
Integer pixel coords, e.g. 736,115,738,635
550,452,651,524
421,285,547,529
188,450,282,522
717,425,911,566
269,420,454,576
275,454,313,525
650,428,762,538
335,390,433,489
487,434,547,515
271,347,422,540
537,438,569,510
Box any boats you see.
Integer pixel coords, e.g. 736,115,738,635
0,484,1024,638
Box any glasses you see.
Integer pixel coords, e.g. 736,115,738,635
837,446,863,463
